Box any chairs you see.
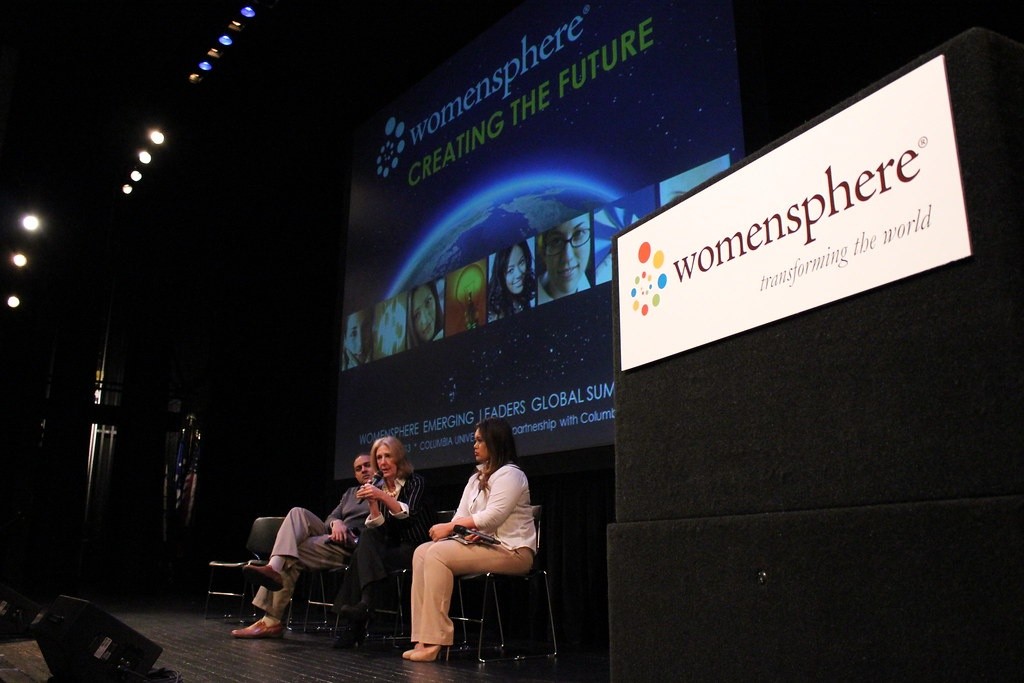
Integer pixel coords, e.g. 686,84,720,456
201,504,559,663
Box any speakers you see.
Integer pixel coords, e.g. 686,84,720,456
29,596,164,683
0,584,43,641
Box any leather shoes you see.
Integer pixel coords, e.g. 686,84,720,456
230,618,284,639
240,564,285,591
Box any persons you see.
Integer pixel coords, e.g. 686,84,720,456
538,214,594,305
231,452,374,638
332,438,424,649
402,419,536,662
487,240,537,321
341,306,374,371
407,283,444,349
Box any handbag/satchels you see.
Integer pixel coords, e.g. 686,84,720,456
322,519,361,552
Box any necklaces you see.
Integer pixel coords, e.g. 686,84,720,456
384,482,397,497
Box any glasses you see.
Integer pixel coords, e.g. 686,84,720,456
543,228,591,256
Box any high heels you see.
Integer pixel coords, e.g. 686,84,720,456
402,642,436,660
341,604,377,629
409,645,452,662
332,624,367,649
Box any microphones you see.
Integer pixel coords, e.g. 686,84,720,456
453,524,501,546
357,470,383,505
